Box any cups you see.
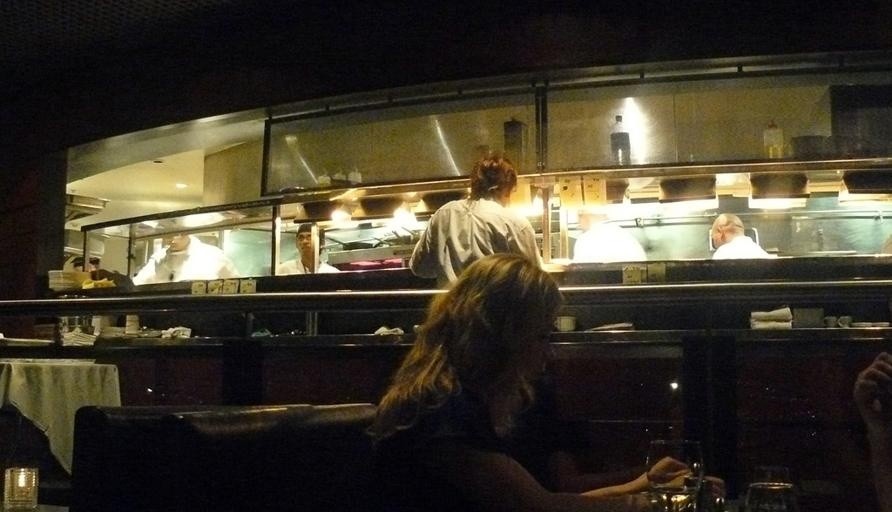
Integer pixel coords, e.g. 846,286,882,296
822,317,850,328
622,262,665,284
127,314,140,333
554,316,576,331
319,172,361,186
6,466,39,511
745,467,803,512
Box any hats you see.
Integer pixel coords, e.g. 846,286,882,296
296,224,325,239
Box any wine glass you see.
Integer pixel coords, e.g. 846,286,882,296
646,439,703,510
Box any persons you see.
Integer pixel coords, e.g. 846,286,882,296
706,213,774,261
408,147,543,291
275,224,341,275
326,253,728,511
852,349,891,511
127,233,242,285
572,207,649,264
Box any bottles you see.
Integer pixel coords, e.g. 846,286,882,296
608,116,631,166
762,120,784,159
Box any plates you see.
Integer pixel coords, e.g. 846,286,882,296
811,251,855,256
137,329,162,336
851,322,889,326
49,270,91,290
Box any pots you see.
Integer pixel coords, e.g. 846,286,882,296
325,237,373,250
385,230,410,244
401,226,426,242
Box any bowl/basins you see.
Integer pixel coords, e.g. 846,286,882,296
57,314,96,336
92,316,119,327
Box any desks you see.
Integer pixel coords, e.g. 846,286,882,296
1,358,121,487
